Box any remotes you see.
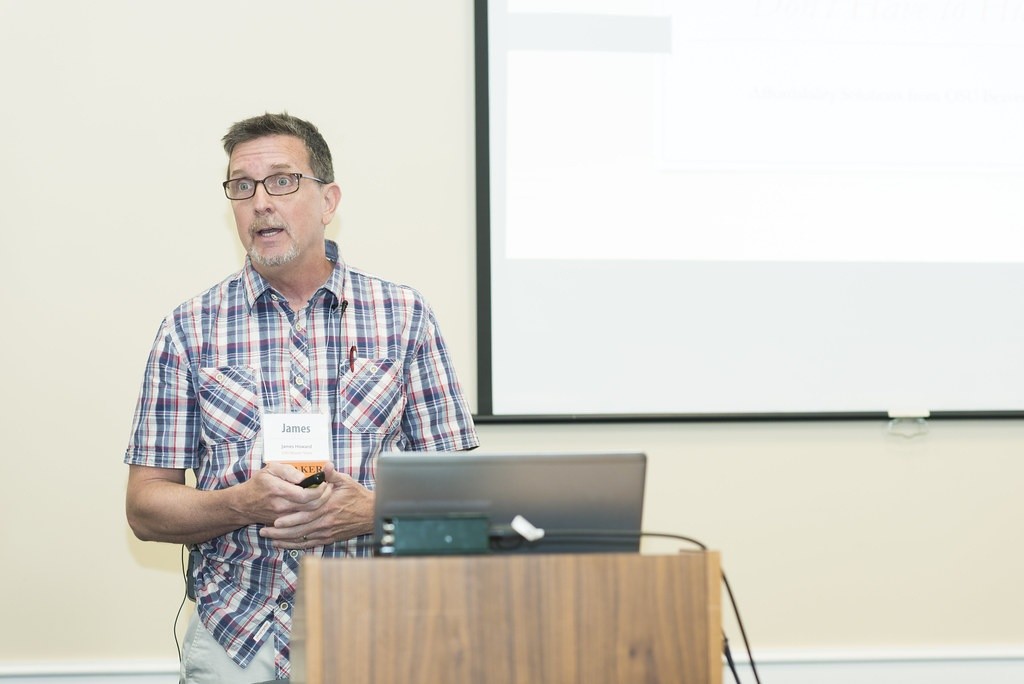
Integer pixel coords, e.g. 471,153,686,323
296,471,325,489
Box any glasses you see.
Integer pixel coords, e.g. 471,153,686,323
222,172,325,201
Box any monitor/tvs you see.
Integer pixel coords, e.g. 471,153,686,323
373,452,646,559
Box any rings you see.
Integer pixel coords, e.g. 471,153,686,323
303,535,307,540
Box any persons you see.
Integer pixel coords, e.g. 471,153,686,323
125,114,480,684
